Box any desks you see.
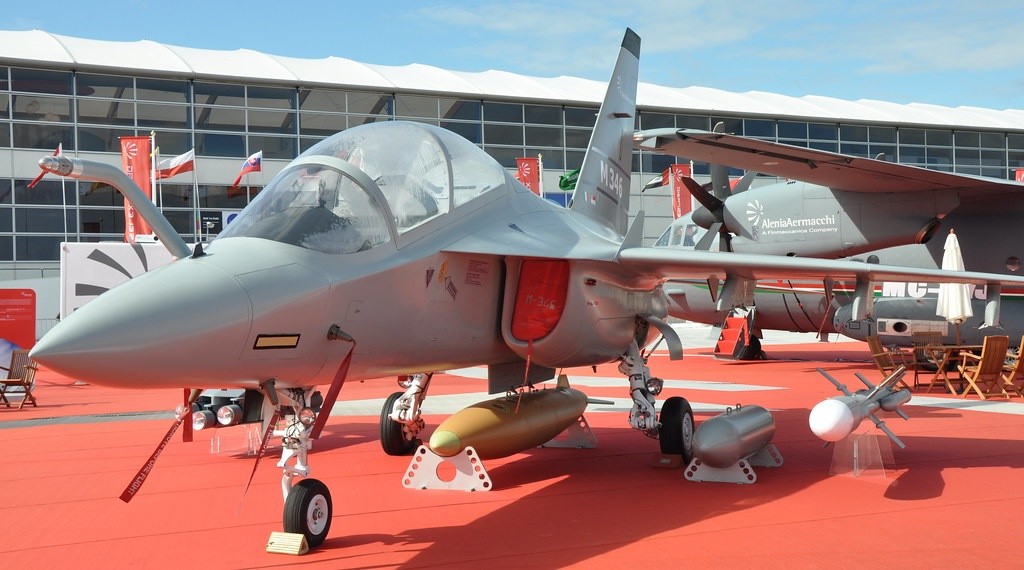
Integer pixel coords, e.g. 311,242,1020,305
916,345,983,395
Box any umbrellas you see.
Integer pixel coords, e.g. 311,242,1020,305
936,234,973,392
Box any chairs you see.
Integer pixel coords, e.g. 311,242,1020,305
957,335,1010,400
1000,336,1024,398
866,336,913,392
0,349,39,409
913,331,948,390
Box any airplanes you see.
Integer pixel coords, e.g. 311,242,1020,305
26,27,1024,554
627,115,1024,373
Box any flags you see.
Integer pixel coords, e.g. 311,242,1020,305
233,151,262,189
559,168,581,190
119,136,151,235
642,169,670,191
156,149,194,179
1015,169,1024,183
671,164,692,220
517,158,539,195
730,176,745,189
